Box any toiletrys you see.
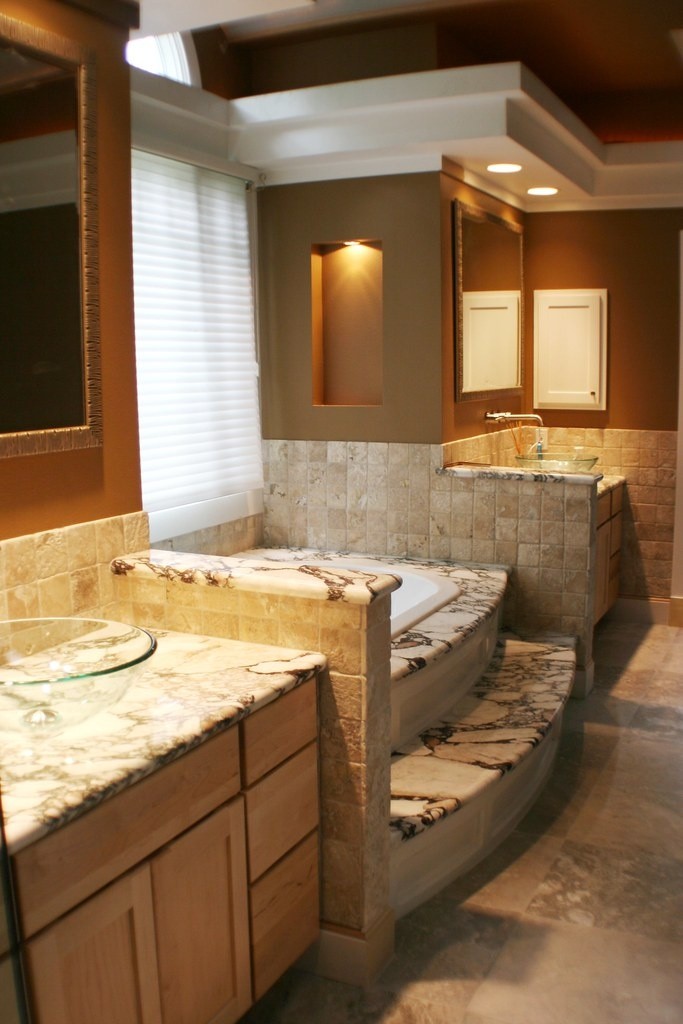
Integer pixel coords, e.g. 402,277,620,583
537,441,542,460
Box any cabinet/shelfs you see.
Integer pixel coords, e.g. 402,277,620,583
0,624,326,1024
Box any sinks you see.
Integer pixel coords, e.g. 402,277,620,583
0,617,158,738
307,560,461,641
514,452,599,472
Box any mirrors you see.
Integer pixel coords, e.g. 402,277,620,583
455,198,526,404
0,14,106,460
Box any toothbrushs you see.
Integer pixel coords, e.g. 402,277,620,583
526,437,542,459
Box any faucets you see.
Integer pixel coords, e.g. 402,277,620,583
485,410,543,427
